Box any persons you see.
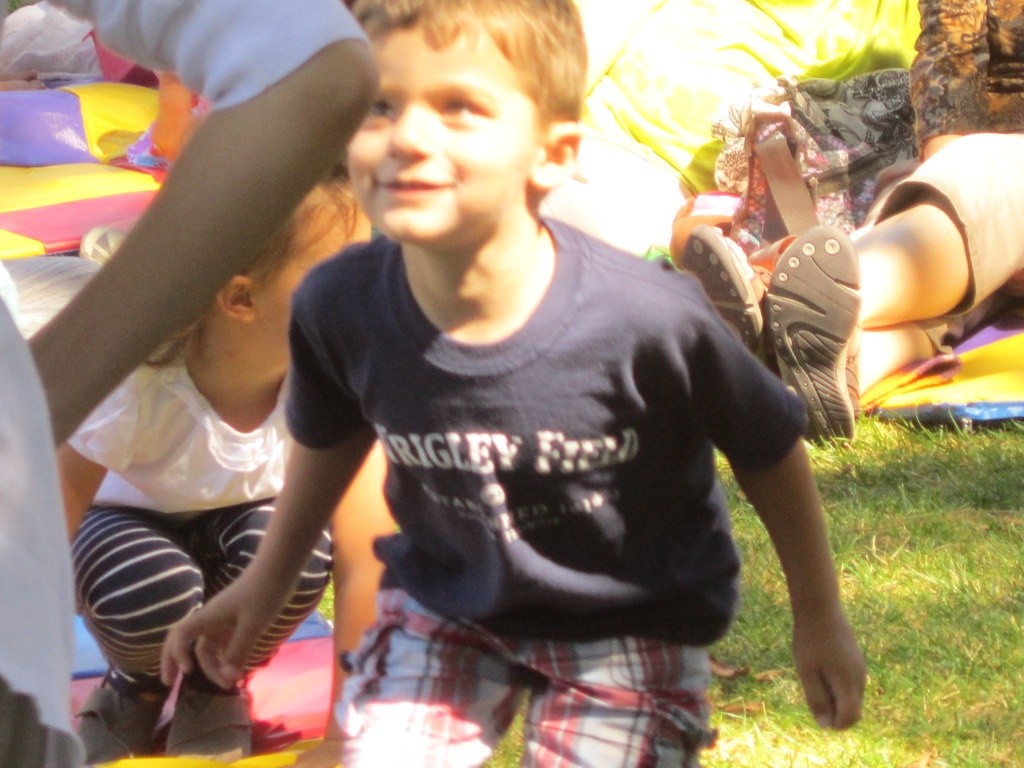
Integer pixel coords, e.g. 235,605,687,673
0,0,385,767
156,0,872,768
57,152,360,767
680,0,1024,453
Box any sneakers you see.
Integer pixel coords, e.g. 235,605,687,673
79,666,162,762
167,677,256,763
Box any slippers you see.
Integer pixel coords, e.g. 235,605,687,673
763,225,861,444
683,225,773,376
